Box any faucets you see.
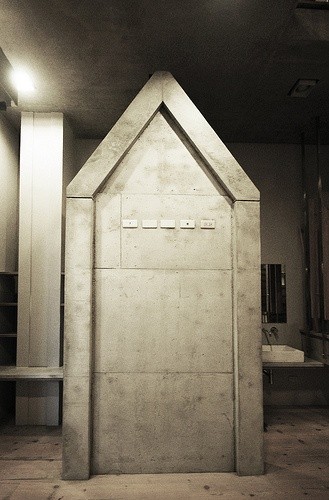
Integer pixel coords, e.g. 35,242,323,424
261,327,272,338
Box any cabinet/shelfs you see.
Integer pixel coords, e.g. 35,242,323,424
0,272,65,339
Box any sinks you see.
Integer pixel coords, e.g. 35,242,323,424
261,342,305,363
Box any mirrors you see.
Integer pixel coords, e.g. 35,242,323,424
261,263,287,323
